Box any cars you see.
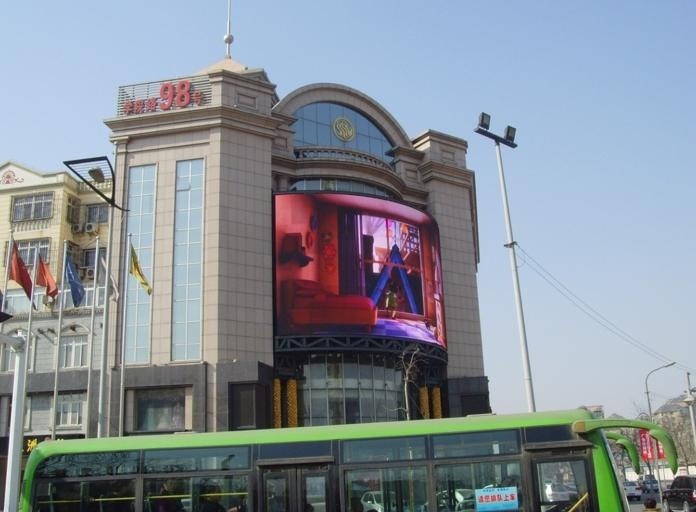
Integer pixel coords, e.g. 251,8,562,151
621,474,658,500
662,475,695,511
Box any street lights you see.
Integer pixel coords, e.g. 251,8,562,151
645,362,677,422
474,111,536,413
63,157,116,435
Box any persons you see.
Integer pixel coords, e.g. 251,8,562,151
42,469,252,512
642,500,661,512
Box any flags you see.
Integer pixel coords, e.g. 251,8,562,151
63,246,85,309
97,249,119,303
35,252,57,312
8,239,37,311
129,244,152,296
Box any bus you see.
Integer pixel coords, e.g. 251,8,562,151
19,406,677,512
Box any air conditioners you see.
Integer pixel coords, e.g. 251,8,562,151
86,269,95,278
72,222,98,233
